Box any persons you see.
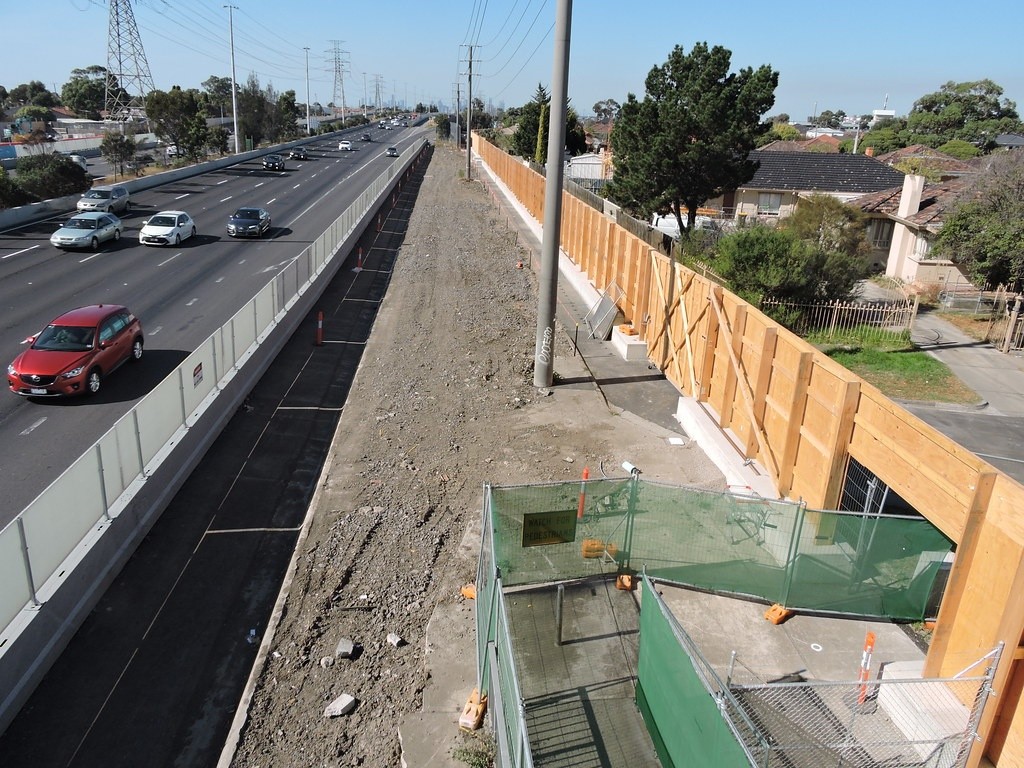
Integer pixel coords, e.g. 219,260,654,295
55,329,78,343
74,221,82,225
89,192,101,198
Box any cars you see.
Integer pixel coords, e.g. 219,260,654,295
361,133,372,142
338,141,352,151
60,152,87,173
50,211,124,252
377,114,417,130
289,146,307,161
226,207,272,239
137,210,197,248
7,303,145,402
385,147,398,157
76,185,132,215
263,155,285,172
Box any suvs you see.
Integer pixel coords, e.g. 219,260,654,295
167,141,187,157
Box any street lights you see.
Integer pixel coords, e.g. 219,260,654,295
303,47,311,136
223,4,241,154
362,72,366,118
